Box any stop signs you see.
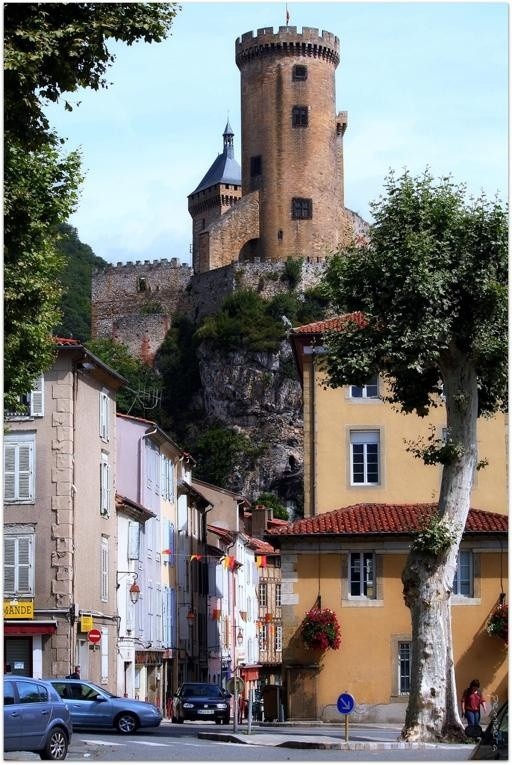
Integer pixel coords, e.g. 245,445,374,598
87,628,101,644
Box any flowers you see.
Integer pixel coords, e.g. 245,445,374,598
484,604,509,651
303,607,341,654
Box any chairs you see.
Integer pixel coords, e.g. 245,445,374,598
21,689,37,703
63,686,83,699
184,689,218,698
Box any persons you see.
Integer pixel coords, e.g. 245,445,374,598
461,679,487,742
66,666,82,696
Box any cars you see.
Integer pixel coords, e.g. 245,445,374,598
4,675,162,760
172,682,231,724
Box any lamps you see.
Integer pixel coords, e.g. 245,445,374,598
116,571,140,605
